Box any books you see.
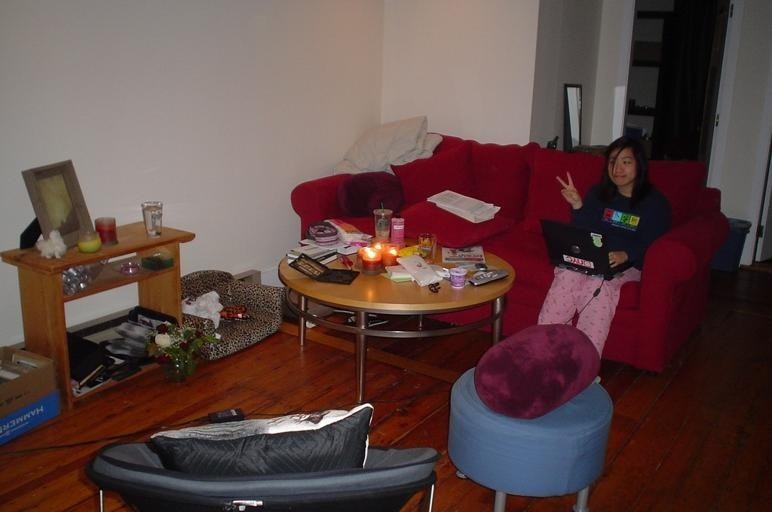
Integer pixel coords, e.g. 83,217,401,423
286,217,373,268
0,346,47,384
426,188,502,224
381,246,487,286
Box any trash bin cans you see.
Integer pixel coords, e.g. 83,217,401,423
711,218,752,272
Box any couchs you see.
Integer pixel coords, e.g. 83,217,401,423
291,133,728,373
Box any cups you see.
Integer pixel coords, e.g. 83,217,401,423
141,200,163,238
450,266,468,290
79,232,102,253
373,208,393,240
417,232,438,264
94,216,118,245
356,239,398,276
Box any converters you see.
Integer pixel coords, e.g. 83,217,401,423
208,408,243,423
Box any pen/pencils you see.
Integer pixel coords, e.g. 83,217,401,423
341,255,352,270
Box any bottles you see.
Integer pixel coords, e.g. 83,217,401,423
391,212,406,247
547,134,559,149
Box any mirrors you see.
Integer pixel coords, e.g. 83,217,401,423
563,84,583,151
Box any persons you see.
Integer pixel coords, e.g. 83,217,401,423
537,135,673,379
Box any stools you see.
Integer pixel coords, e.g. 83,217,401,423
447,366,614,512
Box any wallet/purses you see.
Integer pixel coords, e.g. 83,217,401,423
289,253,359,284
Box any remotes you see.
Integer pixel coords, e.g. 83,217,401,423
468,269,509,286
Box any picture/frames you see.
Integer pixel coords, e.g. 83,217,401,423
22,160,95,251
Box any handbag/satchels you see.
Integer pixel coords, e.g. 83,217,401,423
193,312,219,330
196,291,219,310
306,221,340,246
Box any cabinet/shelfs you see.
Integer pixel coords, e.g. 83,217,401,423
627,10,682,154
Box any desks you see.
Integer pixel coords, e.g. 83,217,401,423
0,221,195,409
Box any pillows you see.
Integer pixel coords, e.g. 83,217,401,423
474,324,599,419
147,404,374,476
346,141,528,247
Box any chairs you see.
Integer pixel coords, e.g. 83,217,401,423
181,270,284,361
86,443,441,512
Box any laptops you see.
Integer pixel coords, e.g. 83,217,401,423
541,219,632,278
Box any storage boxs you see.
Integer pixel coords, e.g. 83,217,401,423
0,391,61,447
0,348,56,417
